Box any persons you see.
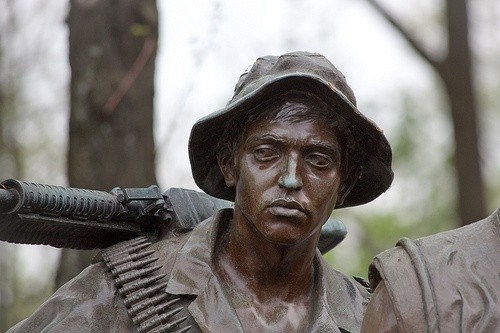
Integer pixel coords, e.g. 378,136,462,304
360,206,500,333
4,51,372,333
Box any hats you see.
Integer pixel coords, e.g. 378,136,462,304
189,51,395,210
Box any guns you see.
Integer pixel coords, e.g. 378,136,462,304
0,176,348,254
